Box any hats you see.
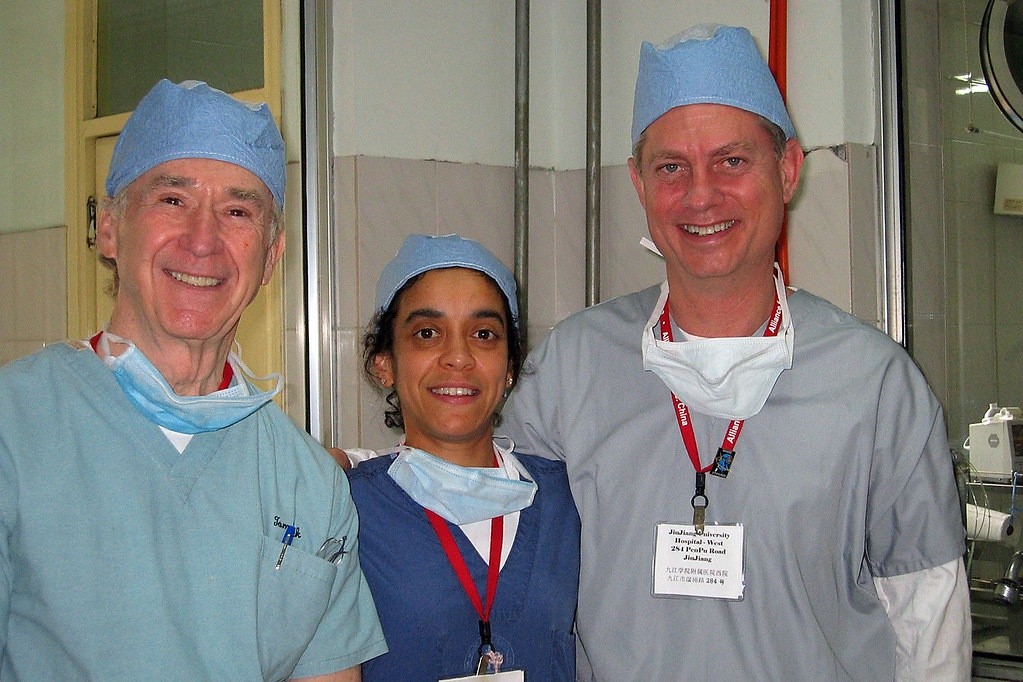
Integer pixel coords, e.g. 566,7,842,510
630,23,797,156
105,79,287,212
372,234,522,351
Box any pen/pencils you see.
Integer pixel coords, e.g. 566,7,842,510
274,526,295,570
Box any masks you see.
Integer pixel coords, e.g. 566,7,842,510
376,434,538,525
640,235,794,420
101,322,285,434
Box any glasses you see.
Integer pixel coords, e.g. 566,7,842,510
315,535,349,565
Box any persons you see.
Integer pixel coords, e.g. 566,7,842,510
345,232,581,682
321,24,973,682
0,77,389,682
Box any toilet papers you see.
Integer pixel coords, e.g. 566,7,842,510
965,503,1020,546
